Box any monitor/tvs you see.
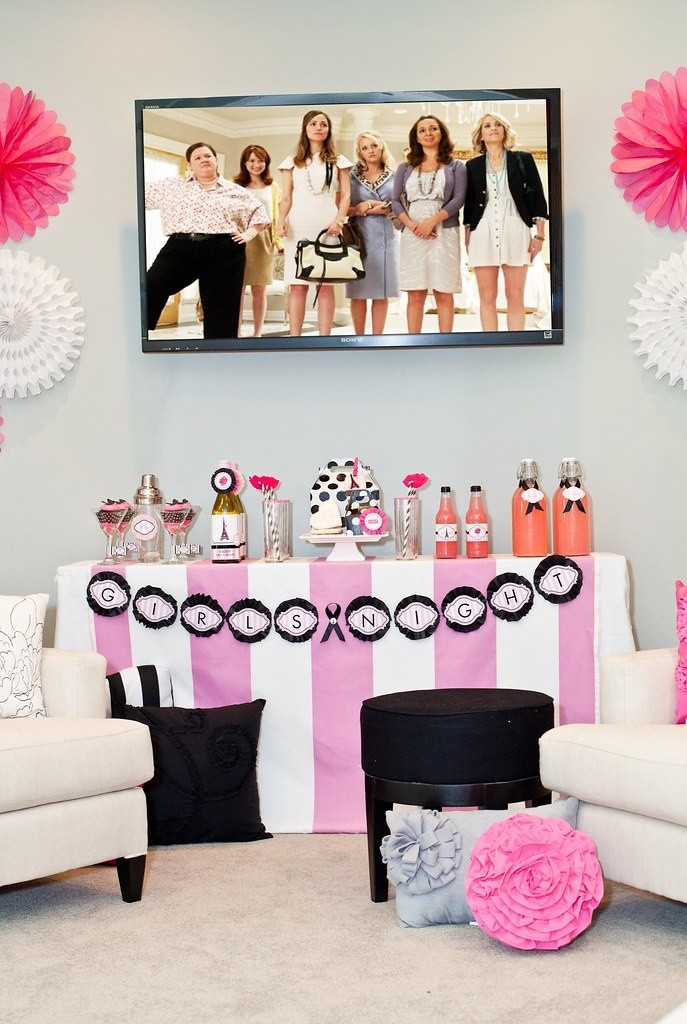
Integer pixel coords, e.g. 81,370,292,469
135,86,563,353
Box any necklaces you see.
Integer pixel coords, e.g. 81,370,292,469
198,177,219,184
417,161,441,196
487,149,506,195
304,162,326,195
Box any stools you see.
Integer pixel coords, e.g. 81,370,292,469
360,688,555,903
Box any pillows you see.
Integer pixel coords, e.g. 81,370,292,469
124,698,275,846
105,655,175,720
0,593,49,718
379,797,578,929
464,813,605,950
675,581,687,725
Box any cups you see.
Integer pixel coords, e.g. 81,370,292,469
132,505,163,563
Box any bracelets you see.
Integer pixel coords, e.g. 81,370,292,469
367,202,372,209
334,219,344,227
534,235,545,241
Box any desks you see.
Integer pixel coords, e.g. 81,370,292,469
55,553,638,836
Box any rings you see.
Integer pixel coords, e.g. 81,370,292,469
333,233,335,236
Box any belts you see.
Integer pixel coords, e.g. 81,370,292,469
171,233,231,242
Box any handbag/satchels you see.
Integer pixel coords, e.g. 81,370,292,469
294,227,366,281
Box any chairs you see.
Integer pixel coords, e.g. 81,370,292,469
538,647,687,905
0,647,156,902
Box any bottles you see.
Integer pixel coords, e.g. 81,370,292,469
466,486,489,557
210,460,246,564
512,459,547,556
435,487,457,559
133,475,163,559
552,458,591,556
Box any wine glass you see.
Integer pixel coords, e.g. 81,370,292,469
154,504,200,564
91,505,136,566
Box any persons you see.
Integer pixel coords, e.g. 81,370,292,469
145,142,273,340
391,114,468,333
275,110,355,336
225,144,284,337
336,130,398,335
465,112,549,331
